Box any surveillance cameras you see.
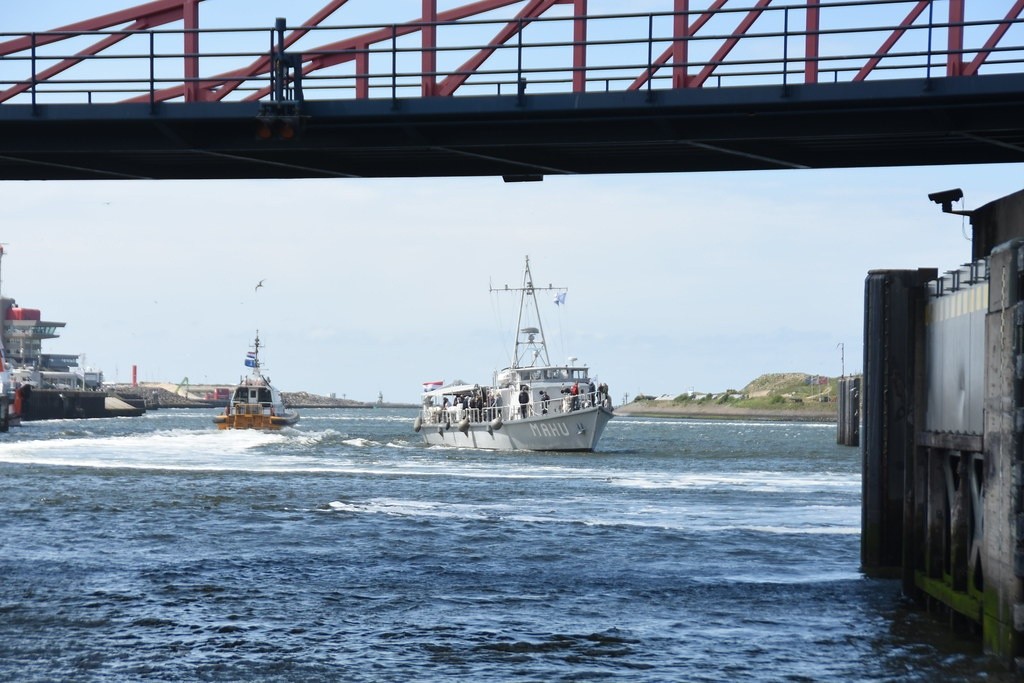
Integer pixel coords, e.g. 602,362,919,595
928,188,963,204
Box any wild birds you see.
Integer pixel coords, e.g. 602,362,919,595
254,279,264,291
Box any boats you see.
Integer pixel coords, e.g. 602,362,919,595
211,328,301,432
415,254,616,454
0,241,161,435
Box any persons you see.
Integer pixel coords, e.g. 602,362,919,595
540,391,551,414
443,394,502,422
588,380,595,406
519,388,529,418
596,382,608,407
571,380,580,410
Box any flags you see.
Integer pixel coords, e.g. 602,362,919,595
553,292,566,305
422,381,443,392
247,352,255,358
245,360,256,367
